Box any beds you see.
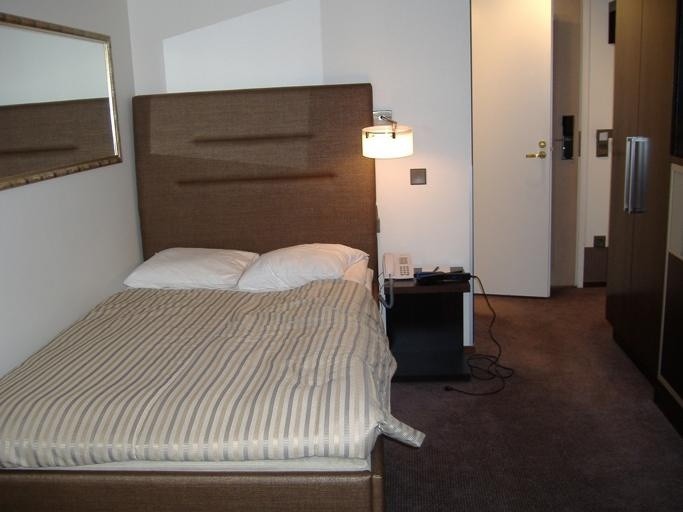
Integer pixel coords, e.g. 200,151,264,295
0,79,400,512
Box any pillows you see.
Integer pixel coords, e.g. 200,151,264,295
121,241,370,292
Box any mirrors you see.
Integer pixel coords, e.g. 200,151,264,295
0,10,124,194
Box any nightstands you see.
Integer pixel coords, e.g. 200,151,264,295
382,267,472,383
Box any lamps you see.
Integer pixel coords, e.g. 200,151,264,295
361,109,415,161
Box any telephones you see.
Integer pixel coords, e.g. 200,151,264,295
383,253,415,280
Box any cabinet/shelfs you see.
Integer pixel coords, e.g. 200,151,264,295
603,1,680,435
